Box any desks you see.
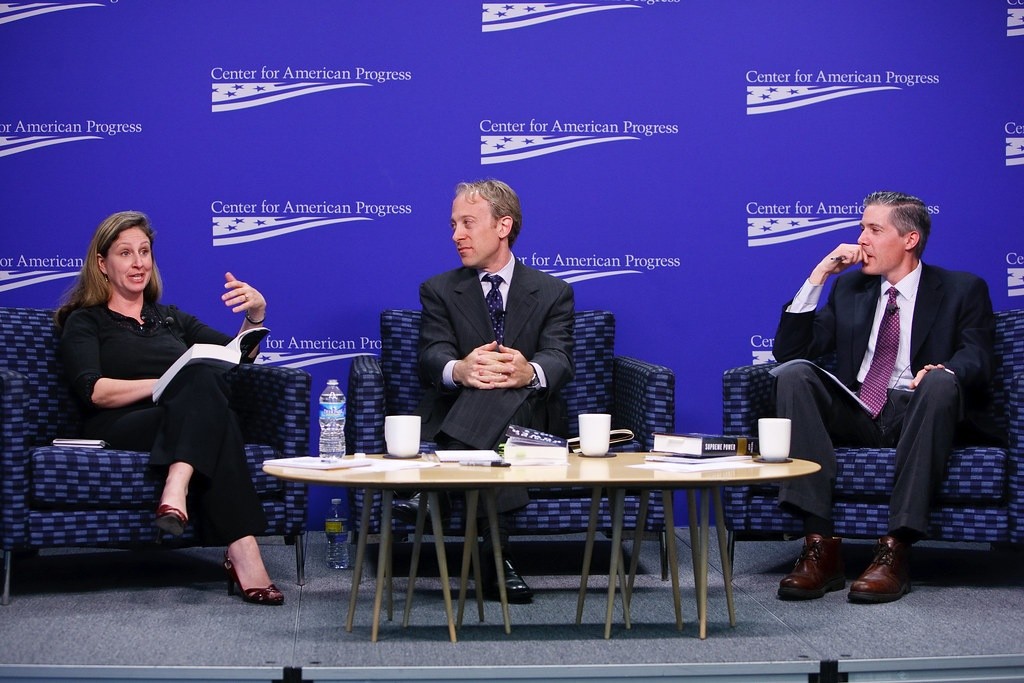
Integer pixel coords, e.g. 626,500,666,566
264,451,822,640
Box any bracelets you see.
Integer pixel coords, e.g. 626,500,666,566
245,311,266,325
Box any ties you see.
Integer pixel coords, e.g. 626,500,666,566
860,287,900,419
482,273,505,346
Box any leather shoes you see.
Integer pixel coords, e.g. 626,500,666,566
505,558,533,597
393,491,450,530
848,535,912,601
779,533,845,597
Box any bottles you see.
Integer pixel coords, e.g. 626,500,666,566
325,498,351,569
319,379,346,460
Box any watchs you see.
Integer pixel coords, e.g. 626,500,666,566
527,373,540,388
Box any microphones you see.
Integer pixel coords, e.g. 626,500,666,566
887,304,895,314
494,308,509,317
164,317,175,327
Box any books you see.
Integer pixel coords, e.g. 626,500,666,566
644,432,753,464
503,424,569,463
434,449,500,464
263,456,372,470
152,327,271,404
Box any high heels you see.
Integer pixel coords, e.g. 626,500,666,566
225,549,284,604
155,501,188,544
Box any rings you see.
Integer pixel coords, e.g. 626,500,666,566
243,294,248,301
499,373,503,376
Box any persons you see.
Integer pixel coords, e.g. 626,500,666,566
54,211,285,605
767,191,996,602
389,178,575,604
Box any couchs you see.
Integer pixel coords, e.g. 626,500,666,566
1,305,312,608
345,310,676,570
717,307,1023,586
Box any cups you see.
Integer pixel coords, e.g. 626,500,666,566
385,414,421,457
757,418,792,462
578,413,611,457
